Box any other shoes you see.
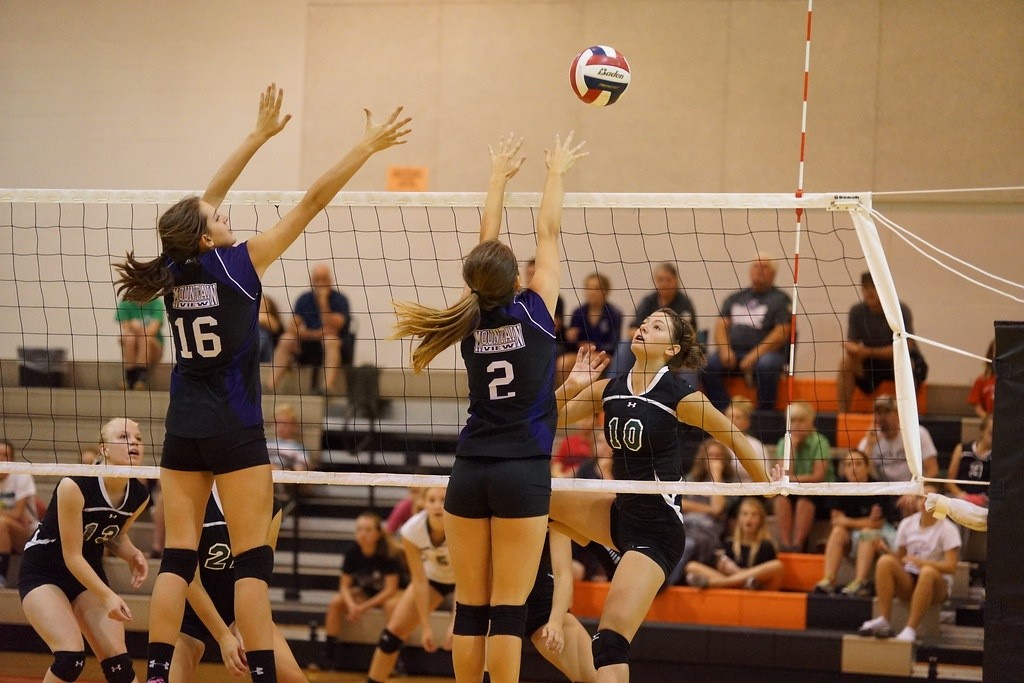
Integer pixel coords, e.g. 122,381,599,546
308,657,339,672
391,661,406,676
133,382,145,390
0,576,9,588
841,580,870,597
144,548,162,559
815,579,835,594
686,572,709,589
779,546,806,553
742,576,758,590
316,389,328,396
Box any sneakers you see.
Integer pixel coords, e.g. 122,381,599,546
889,627,916,643
861,616,892,637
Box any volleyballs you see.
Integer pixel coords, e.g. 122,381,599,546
570,45,632,107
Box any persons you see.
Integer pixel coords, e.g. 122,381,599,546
549,309,785,683
111,82,412,683
168,480,310,683
19,417,150,683
0,252,995,674
367,486,598,683
387,130,590,683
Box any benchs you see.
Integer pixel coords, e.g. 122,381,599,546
0,359,991,683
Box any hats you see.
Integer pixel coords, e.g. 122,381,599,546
874,394,898,408
